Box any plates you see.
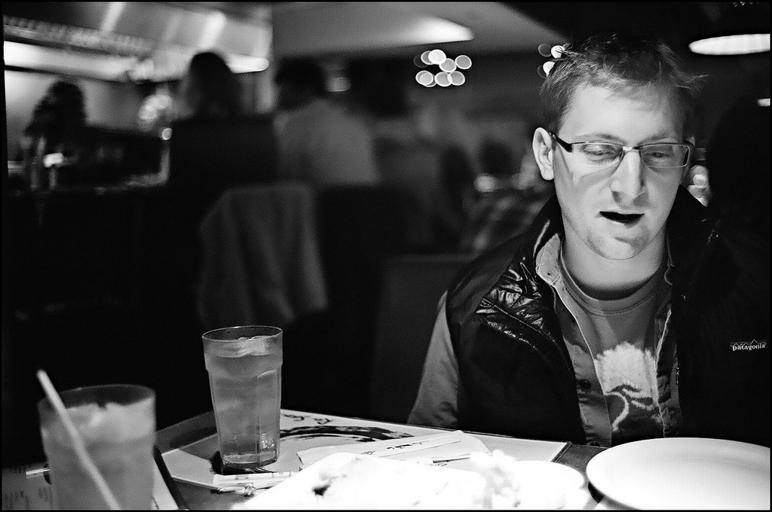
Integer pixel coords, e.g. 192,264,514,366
480,458,586,510
584,435,772,510
230,450,485,511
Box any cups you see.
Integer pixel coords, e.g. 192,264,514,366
201,323,285,470
35,384,159,510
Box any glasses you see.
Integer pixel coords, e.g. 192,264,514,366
548,131,693,169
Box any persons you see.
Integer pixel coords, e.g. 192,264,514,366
406,30,772,446
21,48,513,248
